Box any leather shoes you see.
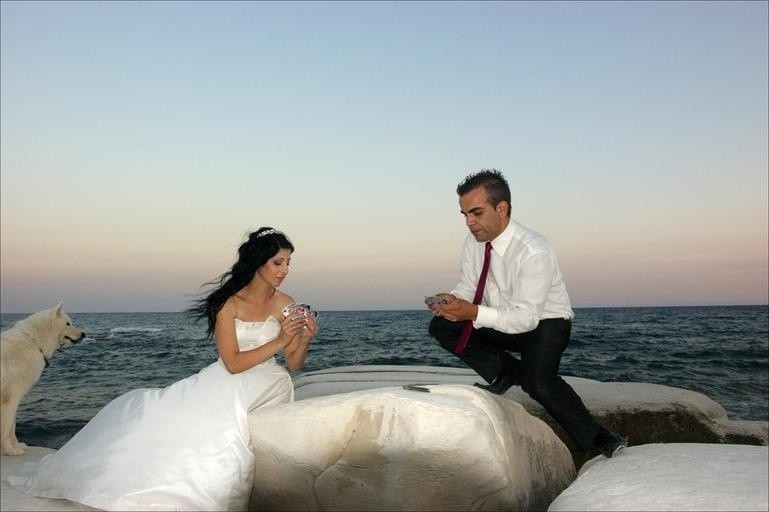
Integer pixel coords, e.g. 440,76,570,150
594,428,629,460
474,359,521,397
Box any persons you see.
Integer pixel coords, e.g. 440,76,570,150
425,165,628,458
0,226,320,512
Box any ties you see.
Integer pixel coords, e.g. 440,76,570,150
453,243,493,356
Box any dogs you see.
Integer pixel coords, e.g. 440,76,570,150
0,301,87,458
